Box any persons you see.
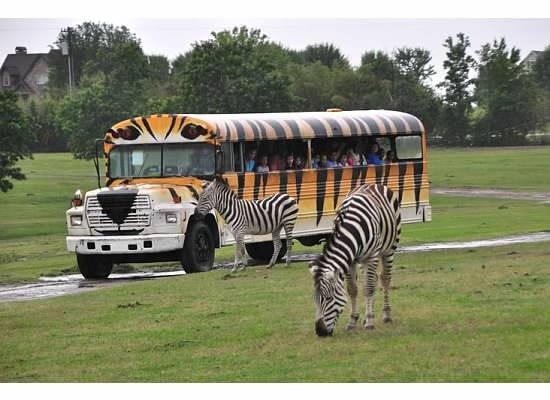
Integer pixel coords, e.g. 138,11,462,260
245,142,396,172
185,152,201,175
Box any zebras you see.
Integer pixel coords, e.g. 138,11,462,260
194,175,300,275
307,181,403,337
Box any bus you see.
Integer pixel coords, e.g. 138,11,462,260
64,107,434,280
